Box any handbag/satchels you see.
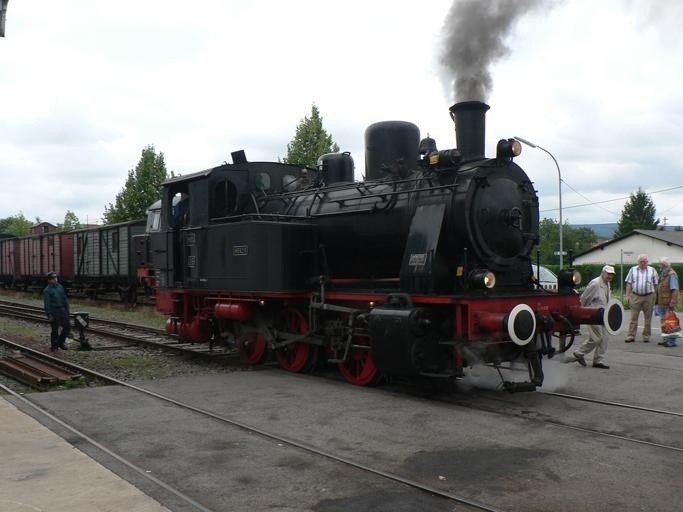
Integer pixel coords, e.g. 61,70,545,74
661,307,681,334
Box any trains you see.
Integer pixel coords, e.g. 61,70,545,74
0,100,625,392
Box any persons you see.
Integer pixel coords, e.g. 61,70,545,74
42,270,71,352
656,258,680,347
624,254,659,343
572,265,616,369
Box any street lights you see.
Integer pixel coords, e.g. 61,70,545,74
514,136,563,269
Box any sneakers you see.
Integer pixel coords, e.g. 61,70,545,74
624,336,635,342
658,340,677,347
643,336,650,343
50,343,68,353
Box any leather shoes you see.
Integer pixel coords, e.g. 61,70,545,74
573,350,587,367
592,362,609,369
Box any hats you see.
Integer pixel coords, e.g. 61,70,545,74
46,271,58,279
603,265,616,274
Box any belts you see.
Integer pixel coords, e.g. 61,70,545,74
632,292,653,297
